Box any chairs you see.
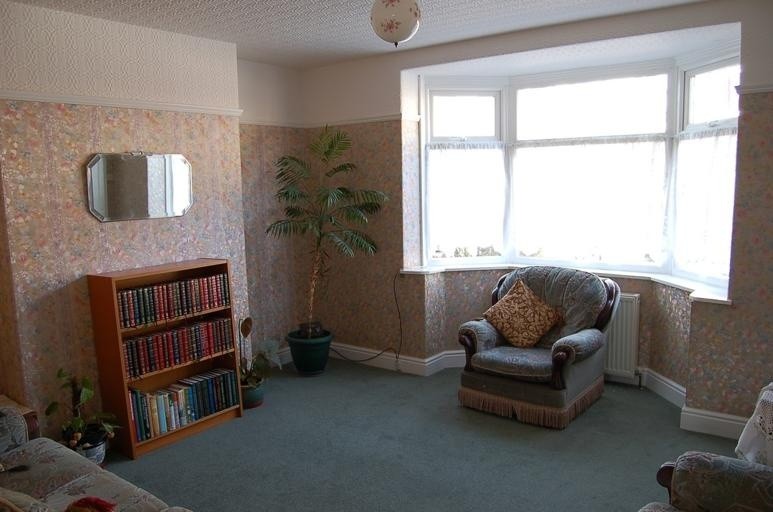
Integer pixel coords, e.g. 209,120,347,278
634,450,773,512
456,265,621,430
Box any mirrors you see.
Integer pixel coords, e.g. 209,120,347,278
85,151,194,223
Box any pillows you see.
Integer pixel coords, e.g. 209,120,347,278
480,278,562,348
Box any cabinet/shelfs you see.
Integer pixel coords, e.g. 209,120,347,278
88,258,244,461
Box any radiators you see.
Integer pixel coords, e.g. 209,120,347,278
604,292,640,380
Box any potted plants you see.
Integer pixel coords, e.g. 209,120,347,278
263,123,391,378
44,368,126,467
238,317,271,409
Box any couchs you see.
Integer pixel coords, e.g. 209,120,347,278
0,407,198,512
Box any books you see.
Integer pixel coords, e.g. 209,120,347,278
117,272,240,442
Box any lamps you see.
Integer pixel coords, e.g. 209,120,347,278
370,0,421,46
